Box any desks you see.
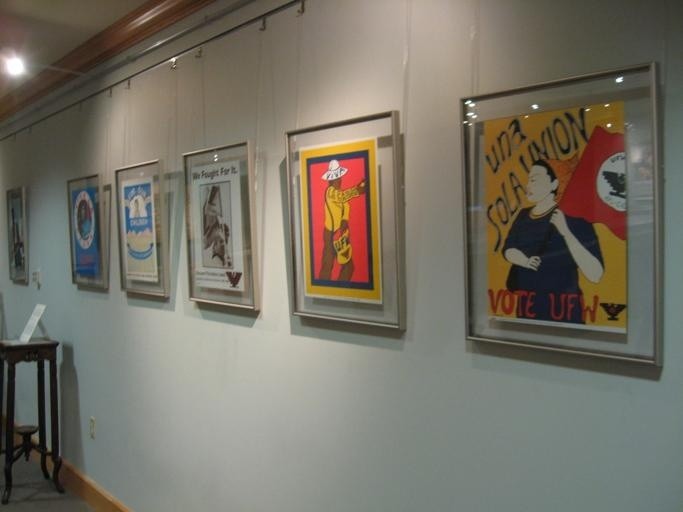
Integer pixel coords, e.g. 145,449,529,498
0,337,65,504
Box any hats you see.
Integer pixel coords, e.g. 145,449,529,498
320,160,348,181
539,151,581,201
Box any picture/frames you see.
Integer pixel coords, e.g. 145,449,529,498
114,158,171,298
4,185,30,284
181,140,263,313
458,62,665,368
66,173,114,290
283,109,406,331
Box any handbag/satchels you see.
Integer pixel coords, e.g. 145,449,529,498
331,228,353,265
